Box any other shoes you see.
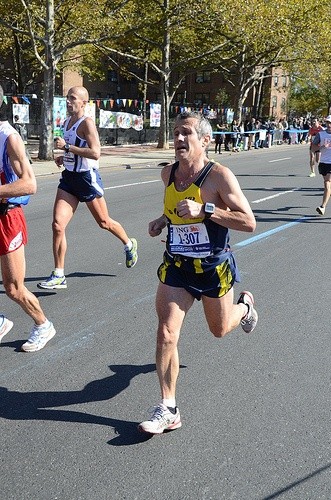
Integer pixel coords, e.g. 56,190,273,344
214,146,264,154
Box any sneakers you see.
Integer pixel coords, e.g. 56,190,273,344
237,290,258,333
0,314,14,341
308,172,315,177
137,403,181,433
37,271,67,288
22,321,56,352
124,238,138,268
316,206,325,215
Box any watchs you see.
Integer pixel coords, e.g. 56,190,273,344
201,203,215,218
64,143,71,152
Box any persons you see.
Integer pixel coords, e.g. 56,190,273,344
136,111,258,433
36,86,138,289
215,115,331,217
0,104,56,352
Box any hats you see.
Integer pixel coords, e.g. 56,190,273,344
324,114,331,122
